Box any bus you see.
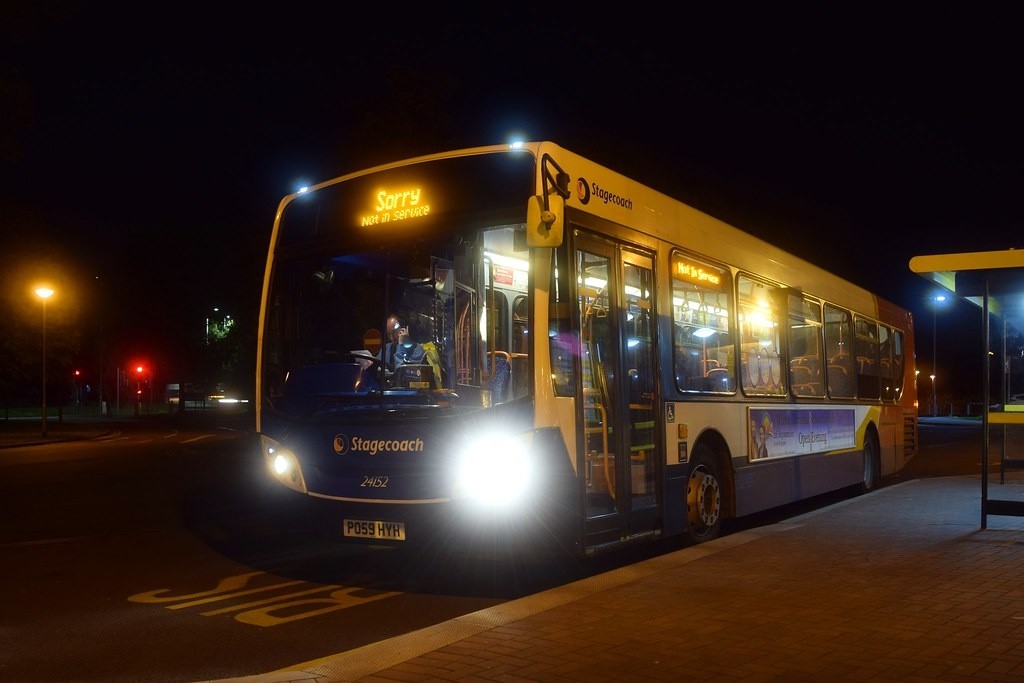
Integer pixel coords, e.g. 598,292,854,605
255,141,917,571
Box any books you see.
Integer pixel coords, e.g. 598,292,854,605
351,350,375,370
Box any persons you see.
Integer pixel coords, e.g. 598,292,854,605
638,321,700,391
357,311,426,372
751,419,769,459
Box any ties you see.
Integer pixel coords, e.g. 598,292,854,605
390,345,396,369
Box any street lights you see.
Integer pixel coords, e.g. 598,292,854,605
32,282,54,438
933,297,945,418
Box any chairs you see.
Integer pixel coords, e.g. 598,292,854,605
479,350,509,407
704,351,899,399
